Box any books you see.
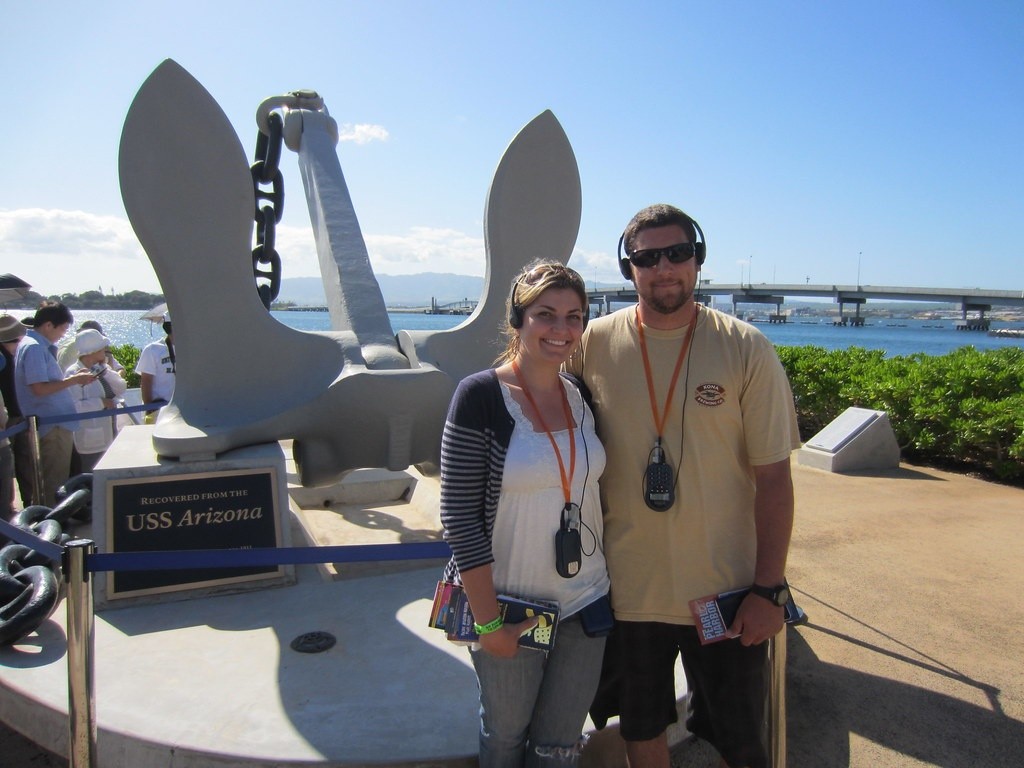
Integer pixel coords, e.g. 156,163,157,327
426,581,561,655
689,576,801,646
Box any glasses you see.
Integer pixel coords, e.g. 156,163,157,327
627,242,696,268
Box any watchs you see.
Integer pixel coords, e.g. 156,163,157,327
750,577,789,608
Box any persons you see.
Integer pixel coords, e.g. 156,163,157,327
560,205,802,768
135,310,176,425
57,321,126,441
64,328,127,520
0,314,36,522
15,303,97,530
439,260,611,768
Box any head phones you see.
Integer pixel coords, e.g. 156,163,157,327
618,217,707,280
508,275,590,334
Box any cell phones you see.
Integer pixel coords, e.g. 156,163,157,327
714,589,791,640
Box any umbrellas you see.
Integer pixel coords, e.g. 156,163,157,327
0,272,32,312
139,302,169,337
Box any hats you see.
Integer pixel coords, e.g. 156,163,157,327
74,328,111,359
0,313,28,342
162,310,171,323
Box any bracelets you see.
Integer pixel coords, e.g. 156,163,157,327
473,615,503,635
